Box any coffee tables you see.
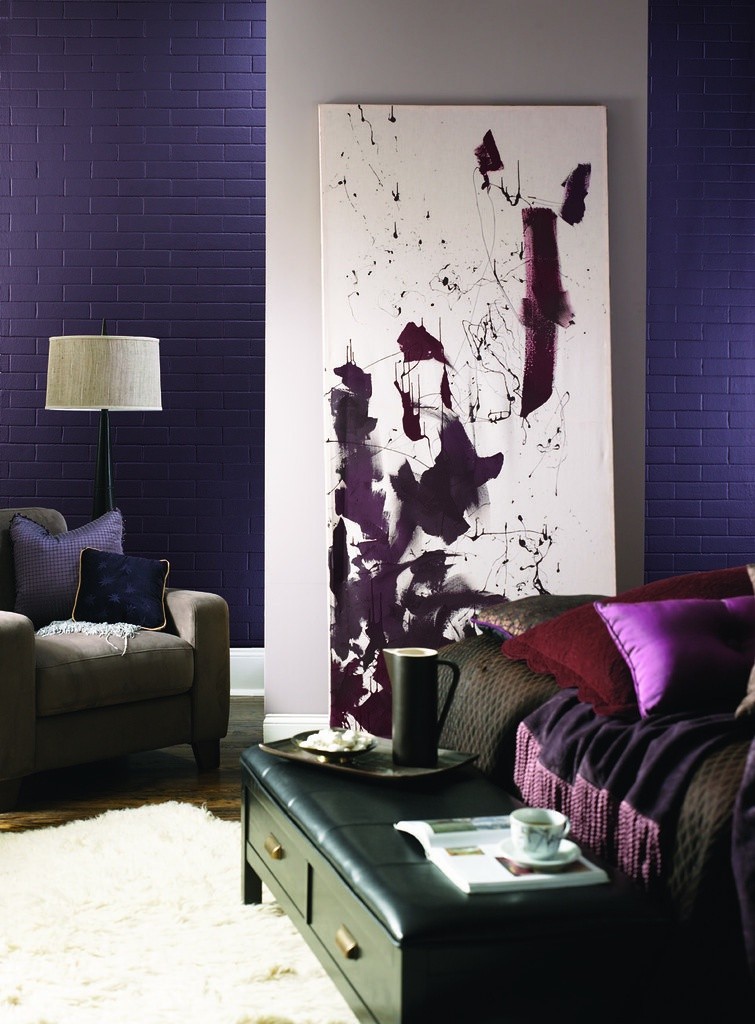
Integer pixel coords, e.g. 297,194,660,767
242,731,655,1024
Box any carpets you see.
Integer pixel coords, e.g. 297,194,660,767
0,802,362,1024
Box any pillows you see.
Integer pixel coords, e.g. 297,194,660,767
590,595,755,724
73,547,168,631
476,596,620,634
10,511,122,631
504,564,755,722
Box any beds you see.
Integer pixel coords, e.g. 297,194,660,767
420,636,755,1011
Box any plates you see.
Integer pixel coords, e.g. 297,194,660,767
290,728,376,757
498,835,580,871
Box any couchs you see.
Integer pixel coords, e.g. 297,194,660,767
0,508,229,810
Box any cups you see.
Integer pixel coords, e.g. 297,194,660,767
509,807,571,862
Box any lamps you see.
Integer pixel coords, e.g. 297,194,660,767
43,317,161,521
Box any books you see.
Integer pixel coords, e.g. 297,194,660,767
393,813,613,894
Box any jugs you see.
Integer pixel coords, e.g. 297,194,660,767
382,644,460,768
